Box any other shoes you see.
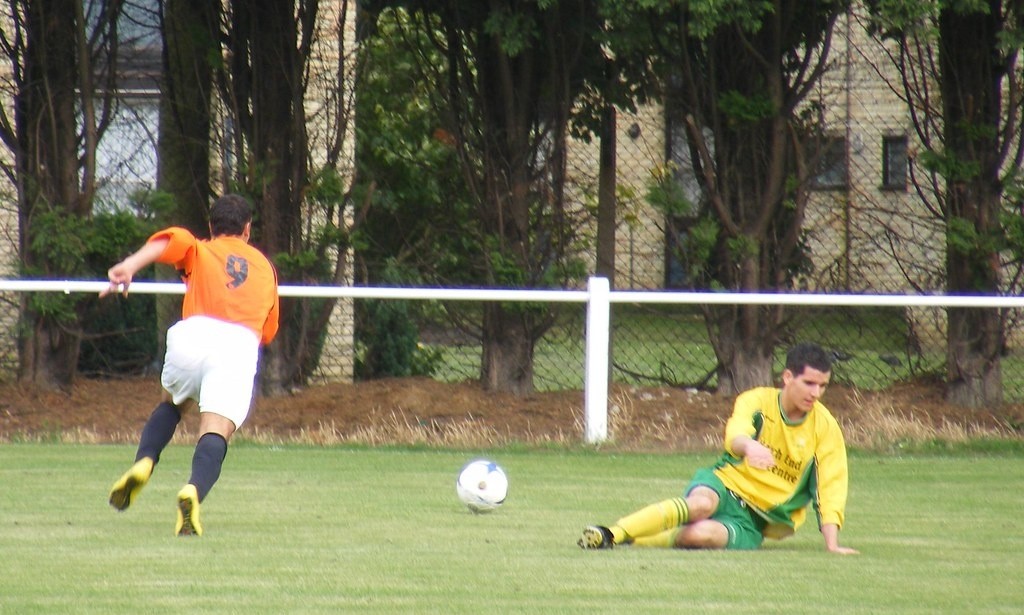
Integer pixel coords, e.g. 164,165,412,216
108,456,154,511
576,525,616,550
174,484,202,537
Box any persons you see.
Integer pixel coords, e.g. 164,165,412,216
98,194,279,537
578,344,859,553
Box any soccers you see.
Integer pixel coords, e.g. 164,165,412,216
455,459,509,511
